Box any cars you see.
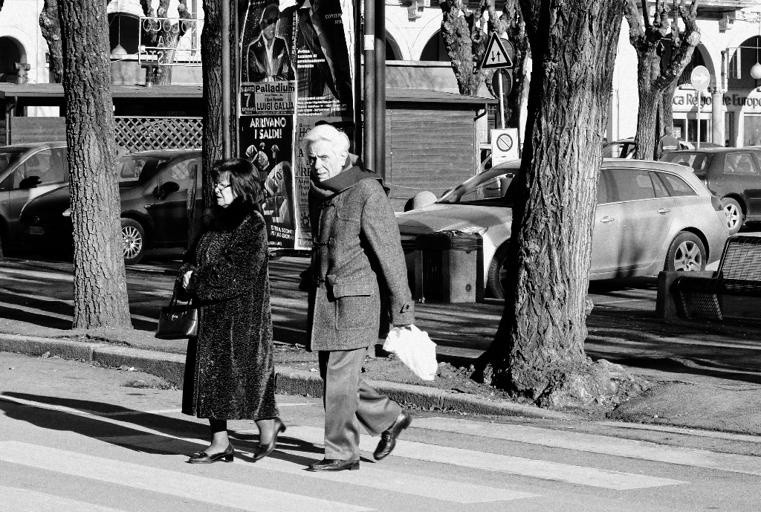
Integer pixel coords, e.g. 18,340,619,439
481,129,745,177
1,139,129,239
19,148,203,263
661,148,761,232
390,153,735,300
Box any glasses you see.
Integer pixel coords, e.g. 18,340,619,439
213,182,230,191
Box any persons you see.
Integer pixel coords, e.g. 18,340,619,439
180,158,286,461
249,8,295,83
657,126,682,163
300,125,415,472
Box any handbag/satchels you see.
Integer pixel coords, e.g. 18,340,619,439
154,305,199,339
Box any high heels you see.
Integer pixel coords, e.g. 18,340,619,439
189,417,286,464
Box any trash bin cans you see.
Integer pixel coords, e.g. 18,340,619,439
417,227,484,306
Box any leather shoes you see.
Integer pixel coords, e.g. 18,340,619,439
308,409,412,472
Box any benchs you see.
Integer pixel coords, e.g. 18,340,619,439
671,234,761,333
